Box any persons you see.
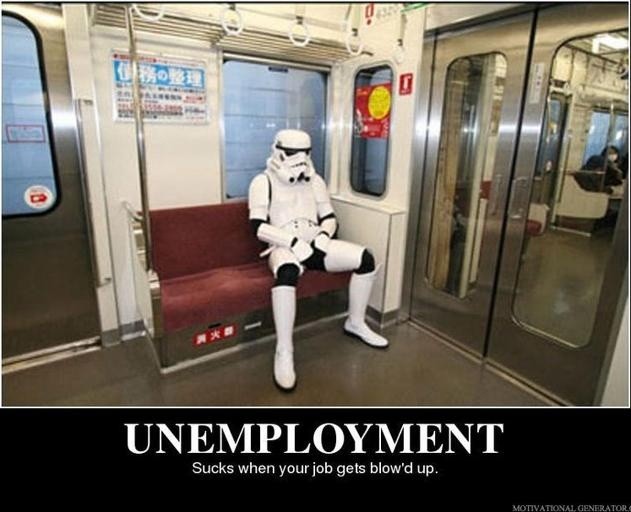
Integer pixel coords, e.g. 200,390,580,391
247,128,389,392
577,147,627,239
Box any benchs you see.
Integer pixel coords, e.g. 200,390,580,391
557,175,626,238
127,200,354,376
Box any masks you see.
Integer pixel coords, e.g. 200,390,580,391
608,153,618,162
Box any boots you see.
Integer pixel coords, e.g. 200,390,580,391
271,285,298,391
343,262,390,350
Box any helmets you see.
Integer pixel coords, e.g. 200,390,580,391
265,128,316,184
